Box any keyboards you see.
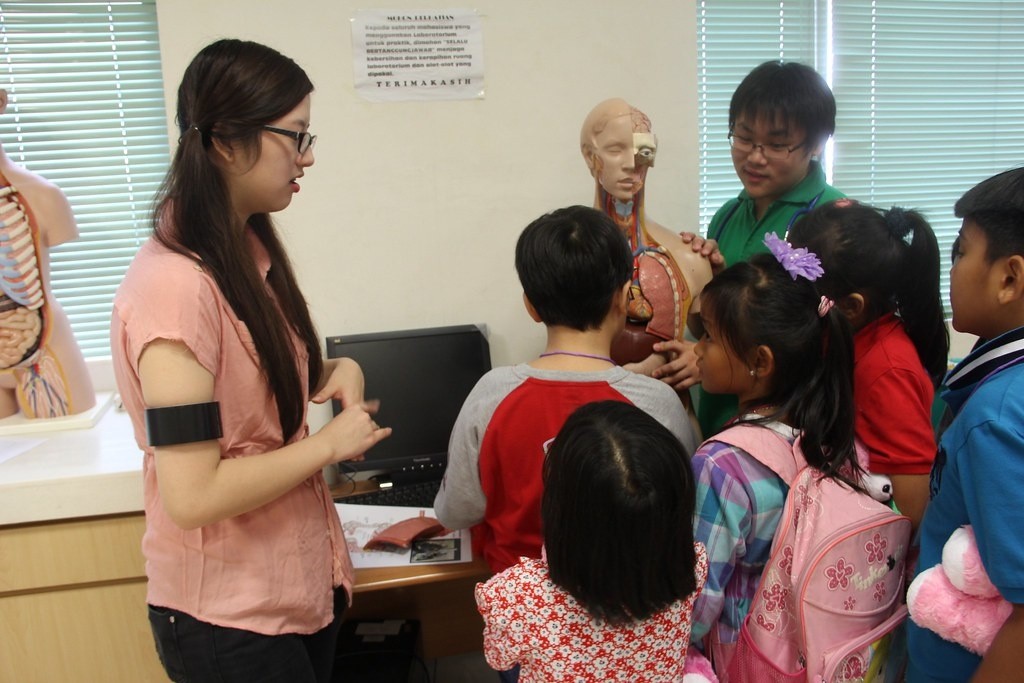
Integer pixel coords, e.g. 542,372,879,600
335,480,442,508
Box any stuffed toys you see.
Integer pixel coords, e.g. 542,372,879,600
683,647,719,683
831,434,893,501
905,523,1013,653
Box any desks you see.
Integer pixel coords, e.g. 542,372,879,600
321,481,493,661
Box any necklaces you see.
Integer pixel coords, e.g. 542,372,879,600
539,352,617,366
750,400,779,413
969,355,1024,394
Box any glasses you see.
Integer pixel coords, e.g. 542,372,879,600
726,125,810,160
259,122,318,152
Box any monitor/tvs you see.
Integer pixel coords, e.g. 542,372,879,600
325,323,492,487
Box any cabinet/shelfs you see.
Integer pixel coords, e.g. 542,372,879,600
0,392,178,683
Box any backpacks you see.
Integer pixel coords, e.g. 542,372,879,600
696,415,912,683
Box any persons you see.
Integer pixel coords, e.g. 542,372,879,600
110,39,393,683
650,60,857,433
787,198,951,523
0,91,95,420
474,400,708,683
430,205,695,578
581,99,713,441
692,233,867,683
900,164,1024,683
